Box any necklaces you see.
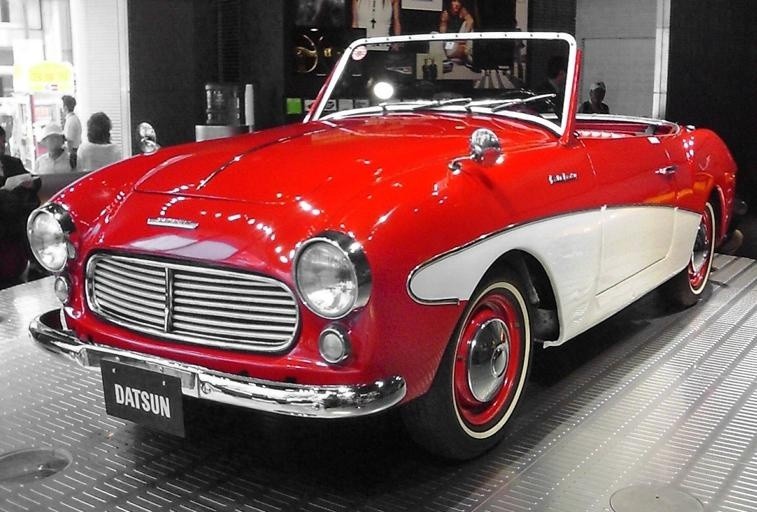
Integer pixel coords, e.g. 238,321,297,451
370,0,376,30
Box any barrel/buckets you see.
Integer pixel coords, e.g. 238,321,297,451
205,82,241,125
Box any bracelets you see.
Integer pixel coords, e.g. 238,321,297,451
458,43,465,46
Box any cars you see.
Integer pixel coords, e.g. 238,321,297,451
24,27,740,463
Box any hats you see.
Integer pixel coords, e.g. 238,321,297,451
590,80,606,92
39,124,64,140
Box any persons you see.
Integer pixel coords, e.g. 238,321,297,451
61,96,83,173
531,55,568,117
578,82,612,113
0,128,30,186
447,1,482,64
32,123,74,172
351,1,403,52
437,0,464,64
76,112,119,172
